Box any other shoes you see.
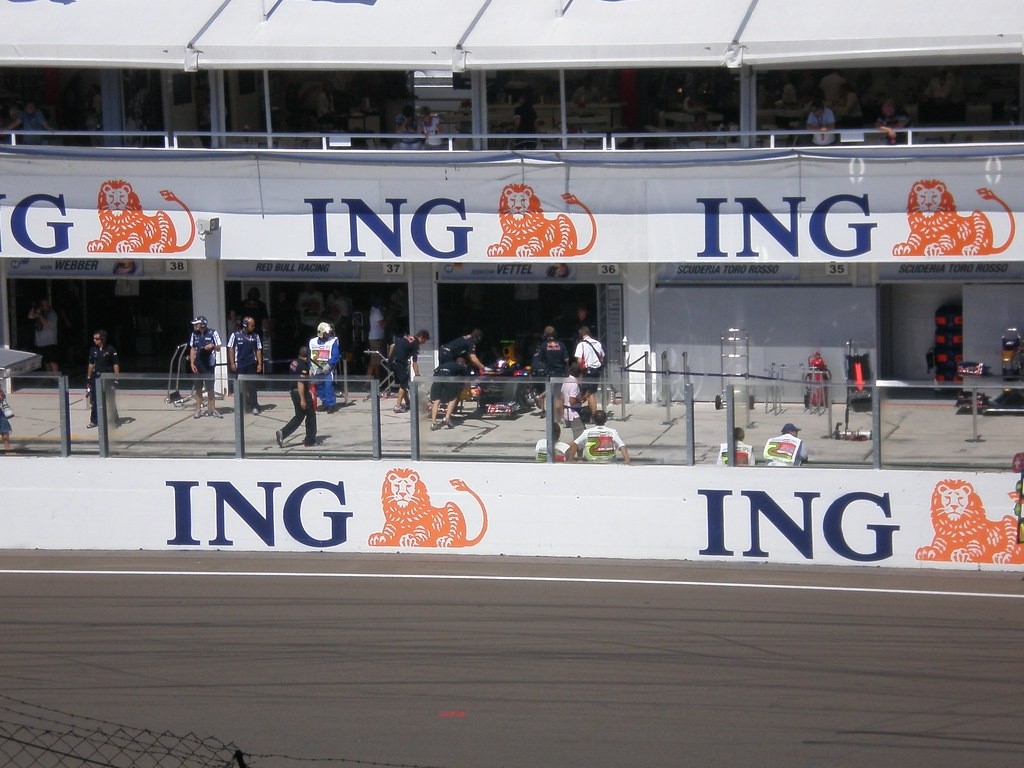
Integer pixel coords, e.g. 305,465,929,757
430,422,442,431
442,417,454,427
318,404,335,413
252,407,259,415
212,410,223,418
194,410,201,418
87,423,97,428
394,402,410,413
276,430,283,447
535,395,542,409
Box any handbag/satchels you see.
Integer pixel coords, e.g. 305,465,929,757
2,399,14,419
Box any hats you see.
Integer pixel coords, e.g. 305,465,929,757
782,424,801,432
191,316,207,324
316,322,332,338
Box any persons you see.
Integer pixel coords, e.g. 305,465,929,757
305,321,340,414
485,71,740,149
189,316,223,418
0,382,12,450
392,106,443,150
387,328,430,413
763,423,809,468
559,362,586,457
756,65,958,145
574,328,605,422
536,423,572,463
28,298,59,383
86,330,120,429
227,316,263,414
570,308,596,345
0,89,105,146
439,329,485,370
315,81,345,129
362,296,392,391
276,346,321,448
717,427,755,466
293,282,353,336
228,286,272,343
569,410,630,463
430,357,467,430
532,326,568,418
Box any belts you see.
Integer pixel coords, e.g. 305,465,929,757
289,388,306,393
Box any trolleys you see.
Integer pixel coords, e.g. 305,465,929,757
714,327,755,409
362,358,410,402
165,343,190,406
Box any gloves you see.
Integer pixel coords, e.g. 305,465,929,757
87,379,92,385
114,380,120,386
322,364,331,373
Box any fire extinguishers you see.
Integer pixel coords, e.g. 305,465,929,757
808,351,824,405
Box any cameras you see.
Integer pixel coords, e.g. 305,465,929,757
34,303,41,310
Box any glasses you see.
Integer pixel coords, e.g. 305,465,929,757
93,338,101,340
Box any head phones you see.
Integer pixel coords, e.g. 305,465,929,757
201,316,206,328
243,317,251,327
101,330,106,342
